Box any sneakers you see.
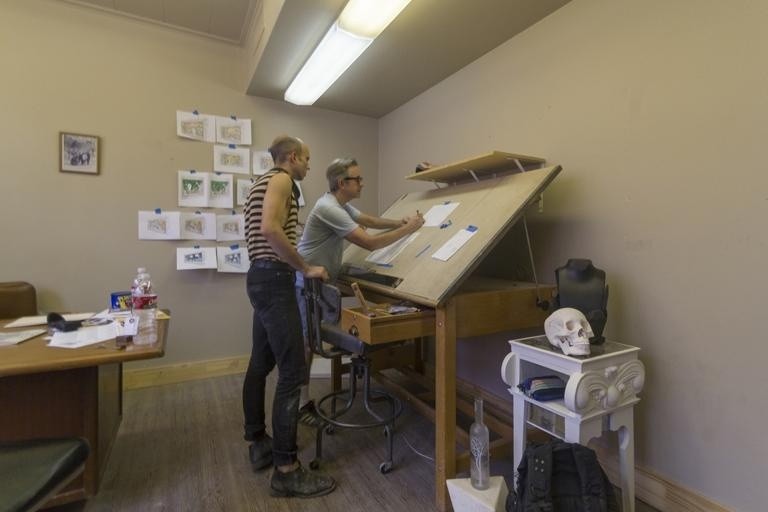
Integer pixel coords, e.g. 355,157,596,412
298,400,336,434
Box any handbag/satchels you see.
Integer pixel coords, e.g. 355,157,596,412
517,376,565,401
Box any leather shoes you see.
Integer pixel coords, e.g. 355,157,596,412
248,435,275,469
270,465,335,497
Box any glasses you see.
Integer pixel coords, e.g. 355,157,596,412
344,176,363,182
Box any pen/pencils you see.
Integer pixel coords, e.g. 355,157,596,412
416,209,419,215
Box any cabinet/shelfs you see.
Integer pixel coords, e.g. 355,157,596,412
300,150,645,512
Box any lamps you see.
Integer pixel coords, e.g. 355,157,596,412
281,0,413,108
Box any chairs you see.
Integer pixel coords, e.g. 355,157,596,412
0,280,38,318
0,435,90,512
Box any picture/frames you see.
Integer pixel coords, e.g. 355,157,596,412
56,130,100,175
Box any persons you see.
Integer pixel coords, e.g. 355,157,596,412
296,158,424,428
239,133,338,500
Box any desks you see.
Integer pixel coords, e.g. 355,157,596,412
0,308,171,500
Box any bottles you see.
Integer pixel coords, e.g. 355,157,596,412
130,267,159,349
468,397,490,491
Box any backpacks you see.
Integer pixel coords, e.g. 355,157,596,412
507,439,619,512
